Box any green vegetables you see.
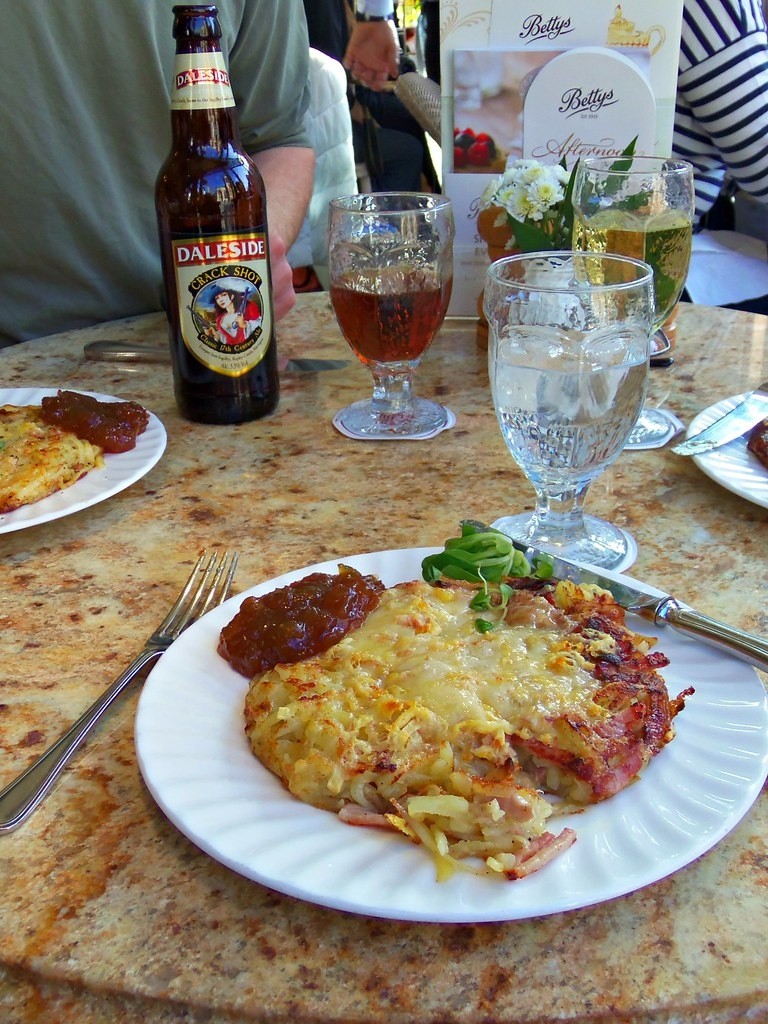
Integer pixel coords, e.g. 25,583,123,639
419,522,553,631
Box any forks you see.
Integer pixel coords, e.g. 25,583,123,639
0,550,241,835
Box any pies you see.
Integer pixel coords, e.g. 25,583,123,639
247,574,690,882
0,403,106,513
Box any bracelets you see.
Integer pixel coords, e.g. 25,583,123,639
356,10,394,22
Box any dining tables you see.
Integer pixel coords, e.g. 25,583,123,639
0,292,768,1023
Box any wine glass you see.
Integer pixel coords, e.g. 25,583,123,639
482,252,655,569
327,192,454,439
572,155,696,444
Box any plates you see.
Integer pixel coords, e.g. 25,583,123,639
686,389,768,508
1,388,167,531
133,545,767,924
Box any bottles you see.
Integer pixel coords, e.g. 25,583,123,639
154,4,280,427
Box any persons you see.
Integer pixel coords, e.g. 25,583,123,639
0,0,445,349
666,0,768,318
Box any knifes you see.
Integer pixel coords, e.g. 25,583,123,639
84,339,354,374
670,381,768,456
459,517,768,673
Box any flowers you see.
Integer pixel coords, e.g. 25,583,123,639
487,133,652,254
